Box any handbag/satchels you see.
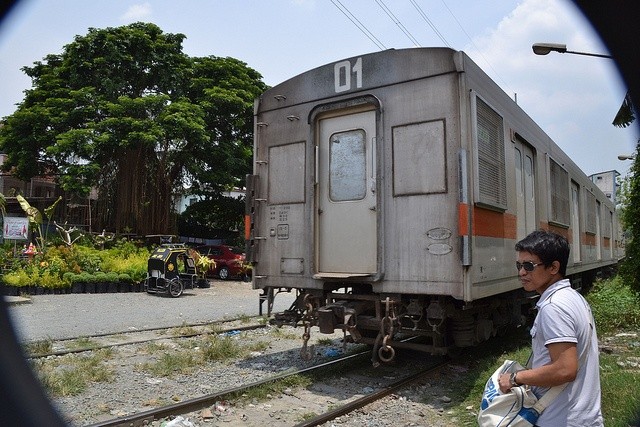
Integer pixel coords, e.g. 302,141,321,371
478,360,539,427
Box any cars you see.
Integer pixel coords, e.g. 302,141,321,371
195,245,245,280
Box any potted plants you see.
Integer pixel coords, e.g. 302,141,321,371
118,274,131,292
196,256,217,288
96,273,108,293
2,266,73,294
106,272,119,292
130,268,146,292
72,275,95,294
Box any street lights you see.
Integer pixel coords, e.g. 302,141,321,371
532,42,613,61
618,154,635,161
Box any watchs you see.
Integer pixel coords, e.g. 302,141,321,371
509,373,520,387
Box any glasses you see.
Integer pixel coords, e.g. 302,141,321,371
516,260,543,272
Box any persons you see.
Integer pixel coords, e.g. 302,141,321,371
498,229,605,427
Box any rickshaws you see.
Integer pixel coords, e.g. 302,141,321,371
144,244,198,298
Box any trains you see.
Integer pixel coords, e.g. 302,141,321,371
242,45,627,367
